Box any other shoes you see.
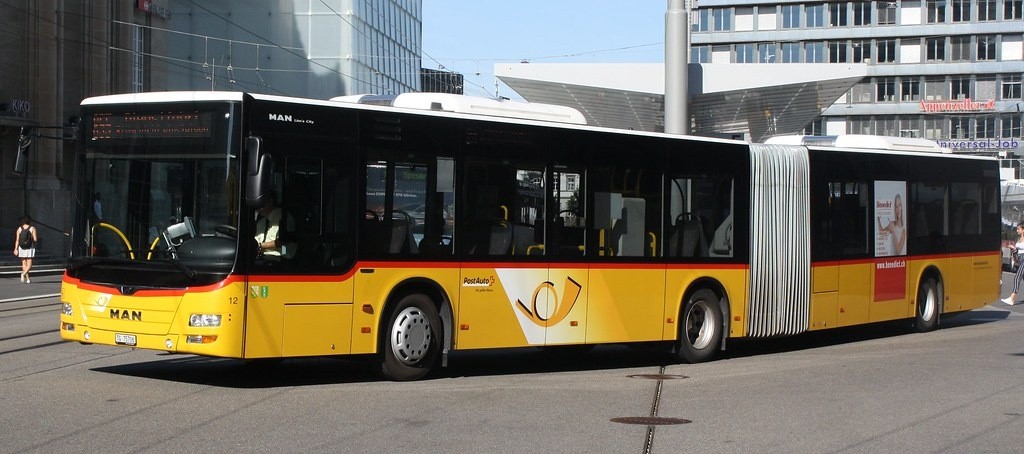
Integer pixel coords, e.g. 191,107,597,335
26,279,30,283
21,273,25,282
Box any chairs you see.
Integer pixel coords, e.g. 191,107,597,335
469,216,513,257
362,208,453,257
666,213,711,258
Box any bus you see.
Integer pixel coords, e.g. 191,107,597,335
15,90,1002,383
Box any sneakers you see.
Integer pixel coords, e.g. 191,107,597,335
1001,297,1013,306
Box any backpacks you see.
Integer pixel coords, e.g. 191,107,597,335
19,226,32,249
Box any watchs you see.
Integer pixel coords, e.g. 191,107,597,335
258,242,264,252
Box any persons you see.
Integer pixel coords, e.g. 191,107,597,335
255,188,297,275
1000,221,1024,307
14,215,38,283
877,195,907,257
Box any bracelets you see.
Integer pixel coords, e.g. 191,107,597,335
1015,249,1018,254
